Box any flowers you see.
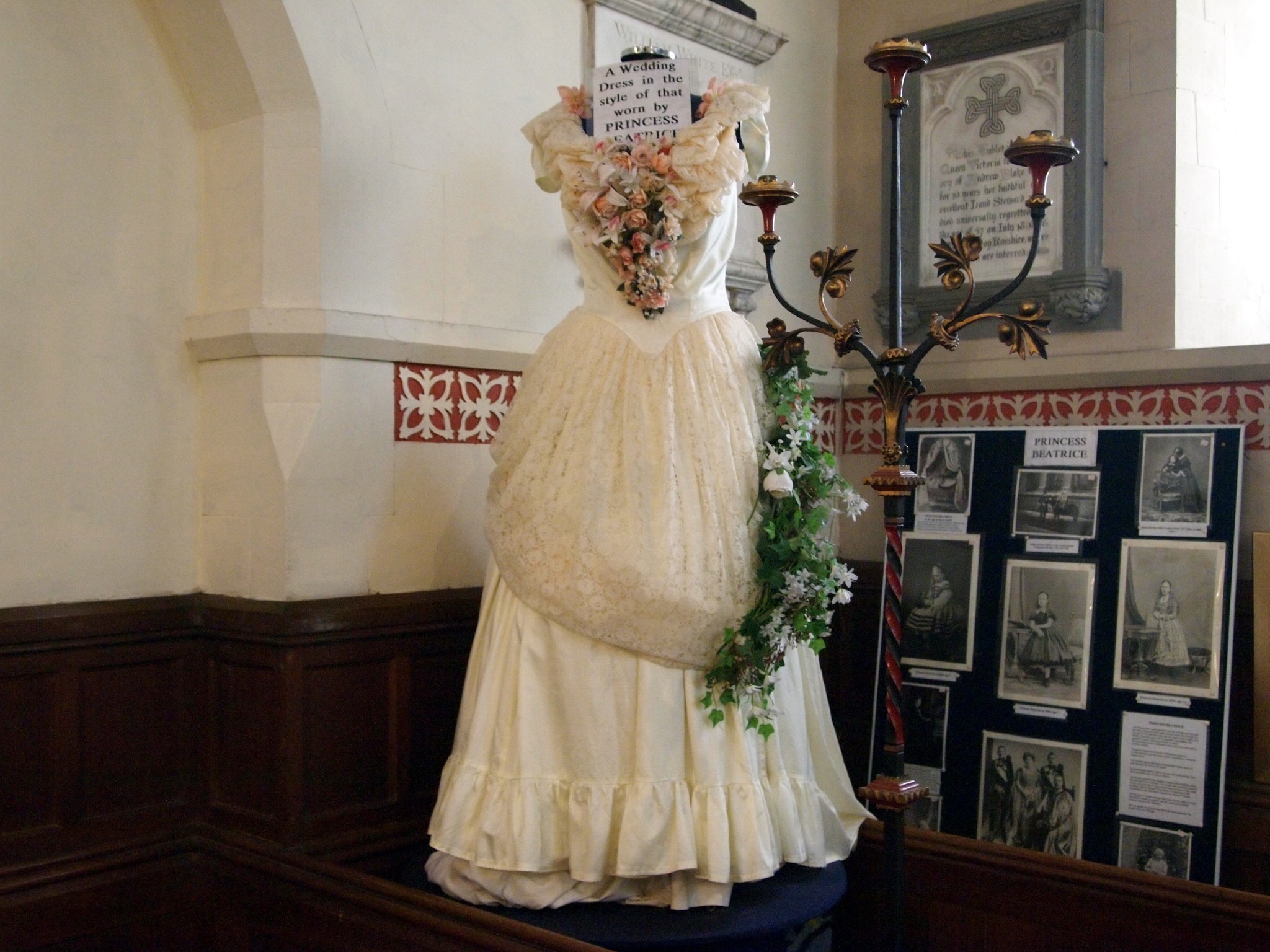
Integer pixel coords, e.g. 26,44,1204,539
705,347,866,739
593,136,682,317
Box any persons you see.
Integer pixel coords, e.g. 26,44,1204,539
421,44,879,911
901,436,1192,877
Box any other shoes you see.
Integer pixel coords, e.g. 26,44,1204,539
1044,677,1050,687
1019,672,1026,682
